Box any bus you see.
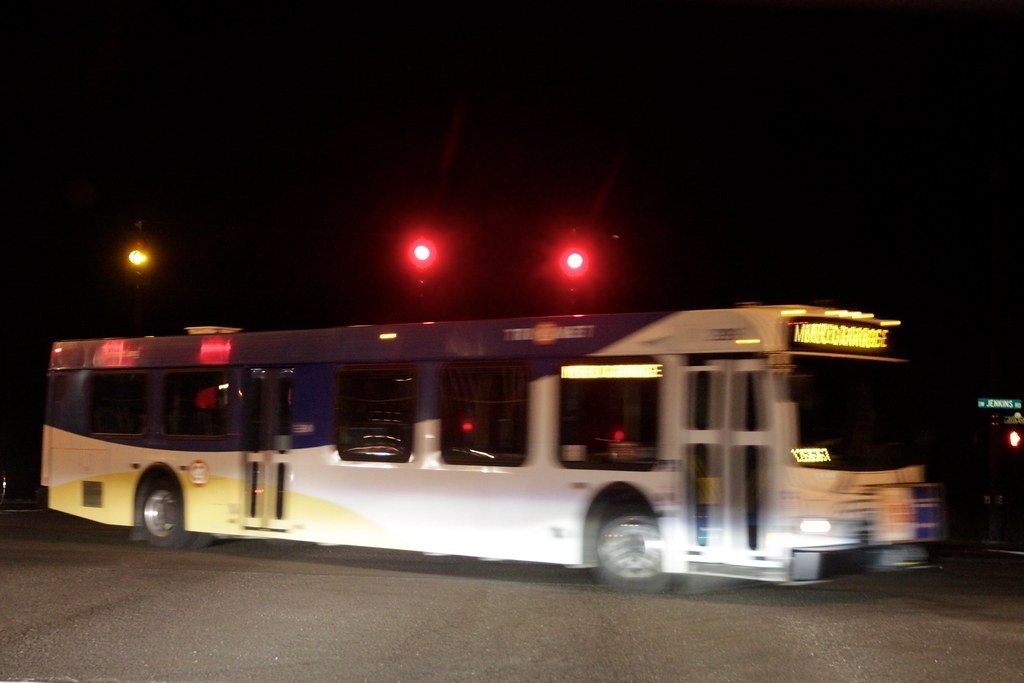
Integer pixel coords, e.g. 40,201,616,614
41,304,950,592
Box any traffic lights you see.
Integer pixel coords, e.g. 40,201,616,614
1007,429,1022,448
